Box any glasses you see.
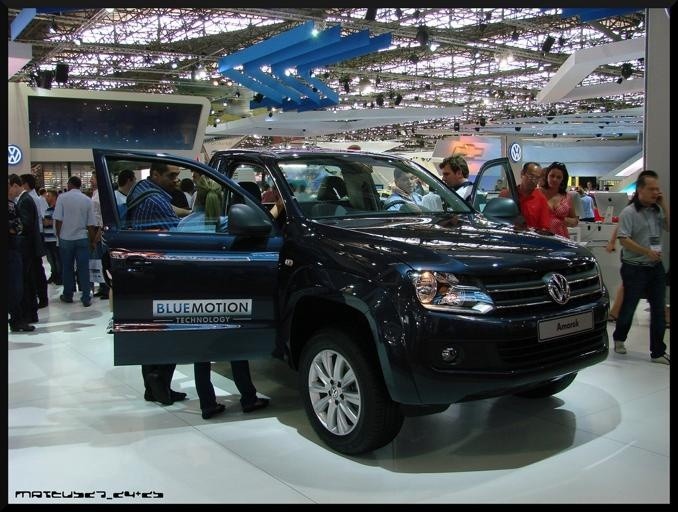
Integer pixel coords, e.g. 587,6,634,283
525,172,539,181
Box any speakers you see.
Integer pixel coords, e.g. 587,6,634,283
542,35,556,52
363,7,378,21
394,94,403,105
255,93,264,103
56,63,68,82
37,70,52,89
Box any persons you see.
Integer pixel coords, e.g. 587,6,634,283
176,173,272,422
604,170,659,323
611,169,671,366
120,160,189,406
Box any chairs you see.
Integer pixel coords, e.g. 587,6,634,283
313,175,355,217
225,179,261,213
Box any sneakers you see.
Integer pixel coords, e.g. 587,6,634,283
10,320,35,333
200,403,226,418
650,352,670,364
613,336,627,355
242,398,270,412
37,275,110,309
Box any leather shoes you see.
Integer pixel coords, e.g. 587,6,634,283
143,388,188,405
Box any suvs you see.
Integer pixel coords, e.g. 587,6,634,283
81,144,616,458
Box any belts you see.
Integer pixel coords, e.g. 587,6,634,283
624,257,665,267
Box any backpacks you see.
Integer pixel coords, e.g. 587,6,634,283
99,202,127,273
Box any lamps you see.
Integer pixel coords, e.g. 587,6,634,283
254,7,645,143
34,62,68,89
